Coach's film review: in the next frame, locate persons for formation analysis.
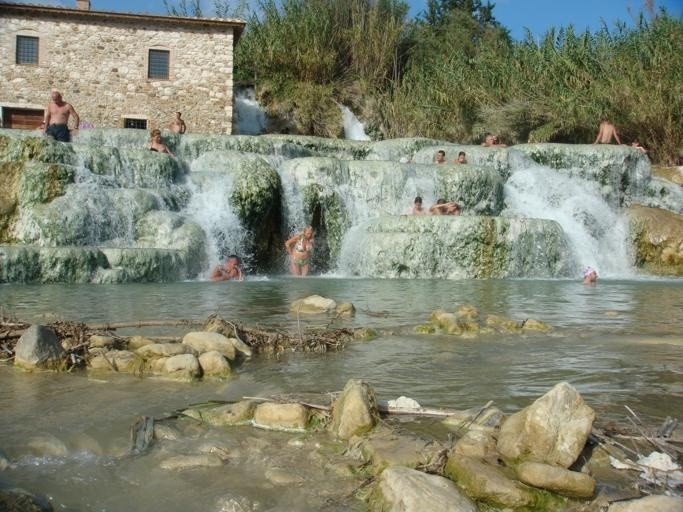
[449,151,466,165]
[583,266,596,284]
[169,112,186,134]
[210,255,242,283]
[434,151,448,165]
[283,226,314,277]
[410,197,426,216]
[43,91,79,142]
[480,132,508,148]
[591,112,621,145]
[629,138,648,154]
[428,198,456,215]
[145,130,176,157]
